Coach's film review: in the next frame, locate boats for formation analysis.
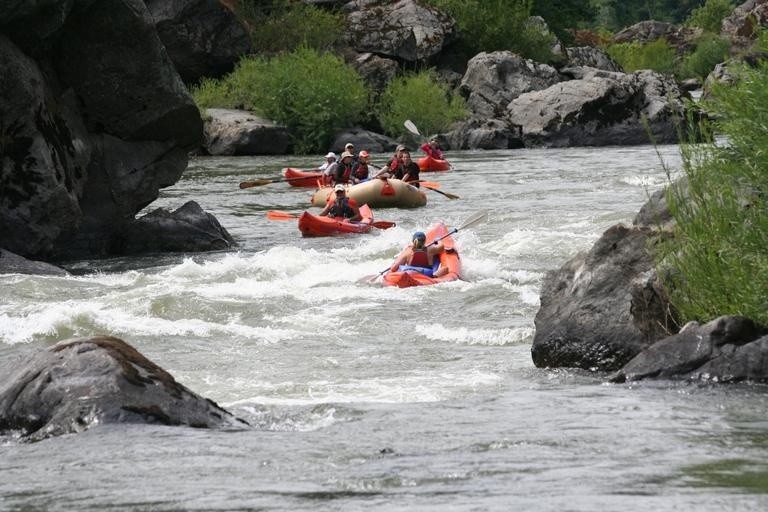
[297,202,372,233]
[284,169,335,187]
[382,222,462,288]
[416,154,450,172]
[309,179,428,211]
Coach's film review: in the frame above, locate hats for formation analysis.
[395,144,406,152]
[324,143,370,163]
[335,184,344,192]
[413,232,425,240]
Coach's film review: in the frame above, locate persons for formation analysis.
[391,232,445,274]
[325,142,419,188]
[421,141,442,161]
[318,184,361,222]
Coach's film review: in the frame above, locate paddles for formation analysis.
[406,181,441,189]
[381,177,395,195]
[368,162,458,198]
[357,209,488,285]
[267,210,396,229]
[404,120,452,168]
[241,174,324,189]
